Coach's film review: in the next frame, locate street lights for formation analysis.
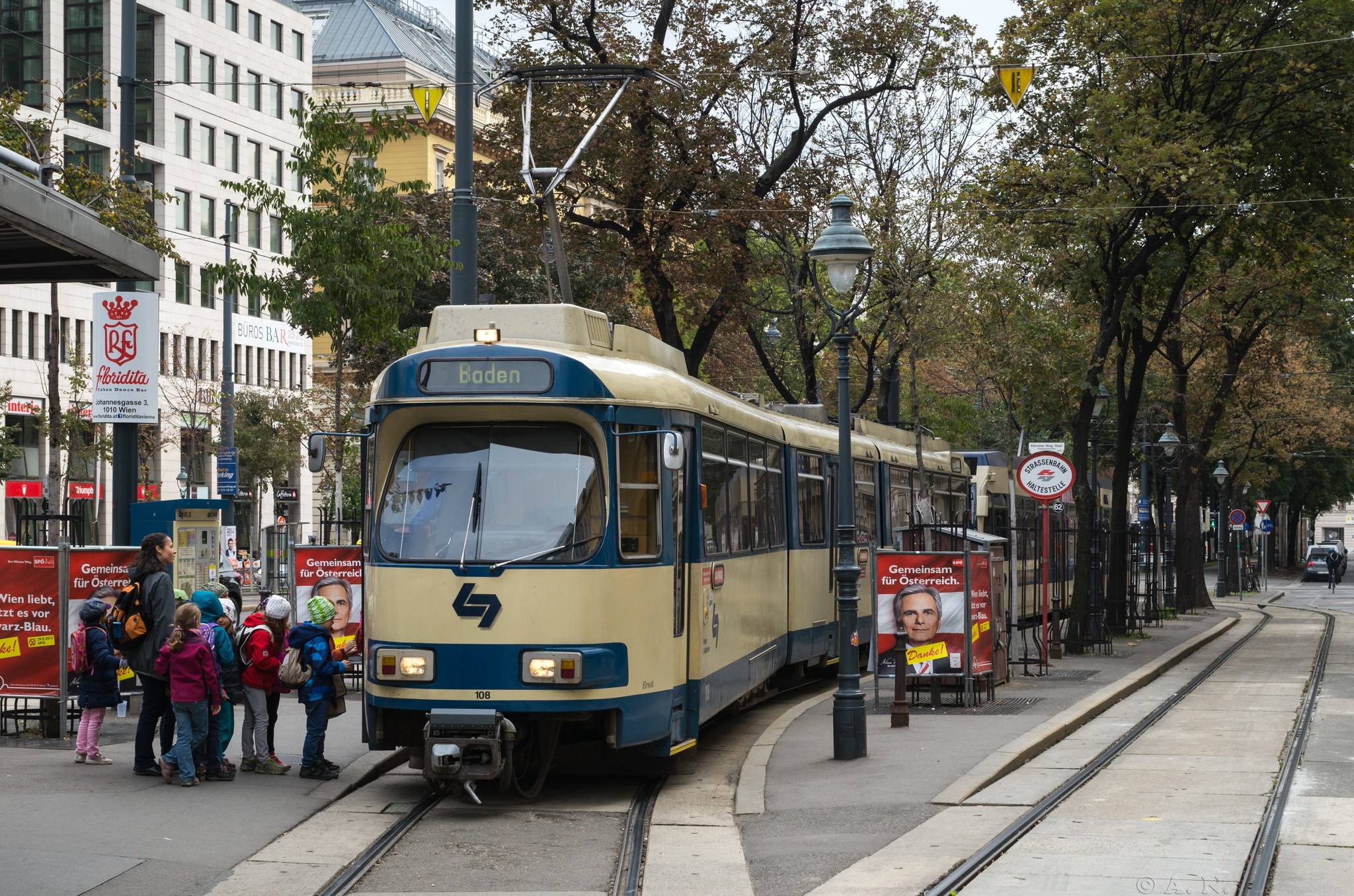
[809,194,878,760]
[1157,422,1181,608]
[1212,460,1230,597]
[1089,377,1111,642]
[1240,480,1252,591]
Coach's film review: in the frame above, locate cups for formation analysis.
[117,701,127,717]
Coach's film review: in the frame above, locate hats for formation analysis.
[79,597,112,620]
[218,598,235,623]
[266,594,291,619]
[201,581,229,598]
[306,595,337,624]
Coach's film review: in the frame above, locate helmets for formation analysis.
[1329,548,1335,552]
[260,597,271,609]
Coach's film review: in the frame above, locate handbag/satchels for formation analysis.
[327,674,346,720]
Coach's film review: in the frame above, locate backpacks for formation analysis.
[105,574,154,650]
[1330,551,1338,562]
[70,622,107,676]
[235,624,274,673]
[200,622,223,681]
[277,638,329,691]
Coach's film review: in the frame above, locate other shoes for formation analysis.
[299,763,339,780]
[135,765,173,777]
[74,753,86,762]
[1328,585,1331,588]
[85,753,113,765]
[317,761,339,773]
[133,761,159,771]
[323,759,340,772]
[181,777,200,787]
[268,750,291,769]
[205,768,235,782]
[222,758,236,767]
[197,762,206,776]
[159,757,174,784]
[220,764,237,773]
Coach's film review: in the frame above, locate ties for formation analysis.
[920,661,931,674]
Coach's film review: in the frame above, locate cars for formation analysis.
[1302,552,1342,583]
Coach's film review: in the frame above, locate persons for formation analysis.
[354,608,362,653]
[73,597,128,765]
[236,595,291,775]
[226,538,235,557]
[878,584,962,675]
[1329,528,1336,539]
[1239,554,1253,581]
[1326,548,1341,589]
[393,450,466,559]
[87,585,121,619]
[127,533,176,776]
[155,603,221,786]
[173,581,244,781]
[286,595,358,780]
[311,575,352,639]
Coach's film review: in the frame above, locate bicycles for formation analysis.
[1244,565,1261,593]
[1330,565,1338,594]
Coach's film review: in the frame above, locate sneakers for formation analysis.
[239,755,256,771]
[254,757,289,774]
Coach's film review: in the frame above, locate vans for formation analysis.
[1306,540,1348,576]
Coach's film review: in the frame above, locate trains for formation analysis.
[307,62,1115,806]
